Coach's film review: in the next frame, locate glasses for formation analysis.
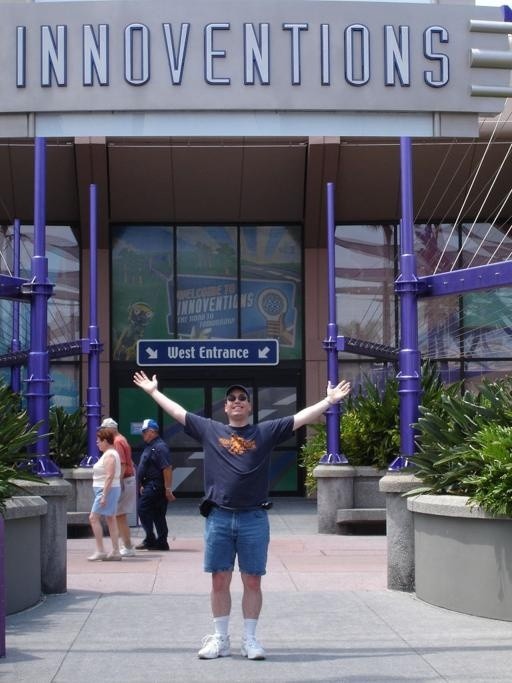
[227,394,248,402]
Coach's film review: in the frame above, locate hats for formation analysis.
[224,384,251,402]
[96,417,118,430]
[139,418,160,432]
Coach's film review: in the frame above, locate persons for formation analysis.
[134,419,176,550]
[101,417,137,557]
[133,369,351,660]
[87,429,122,561]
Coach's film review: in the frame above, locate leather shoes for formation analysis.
[135,539,156,549]
[147,540,170,551]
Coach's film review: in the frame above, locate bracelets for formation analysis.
[102,493,107,497]
[165,488,171,490]
[325,397,333,405]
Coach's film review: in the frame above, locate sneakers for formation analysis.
[196,632,232,658]
[87,552,107,561]
[120,545,136,557]
[102,552,123,562]
[239,635,266,660]
